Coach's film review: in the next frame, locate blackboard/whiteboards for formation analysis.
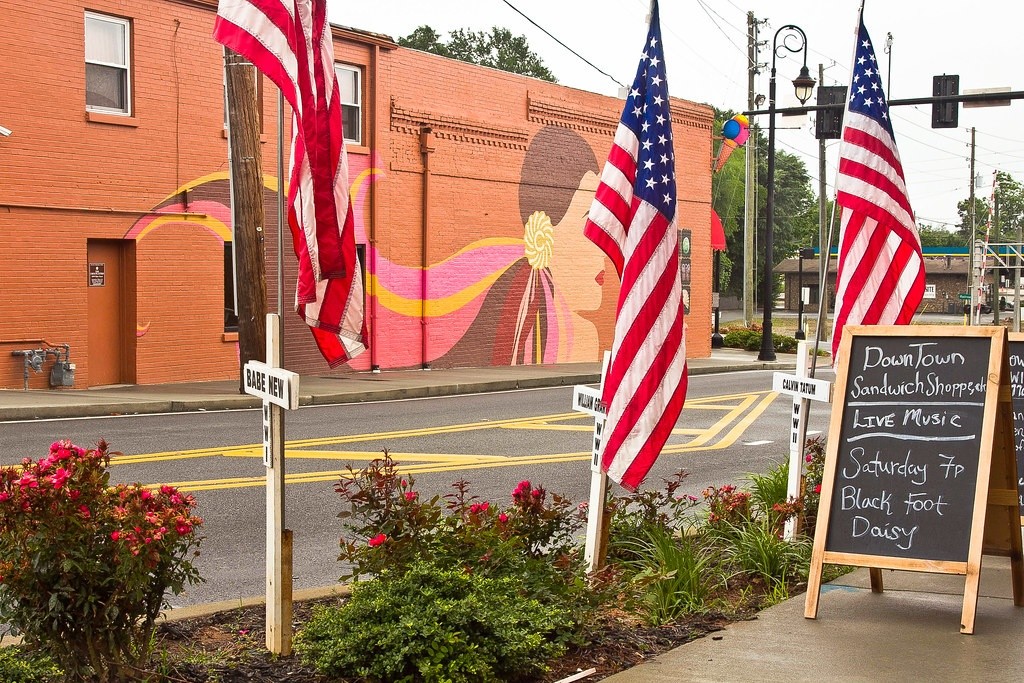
[802,325,1024,634]
[1005,325,1023,534]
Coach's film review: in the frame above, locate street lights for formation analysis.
[755,24,816,361]
[969,171,983,326]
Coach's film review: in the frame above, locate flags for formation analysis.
[213,0,370,370]
[584,0,691,495]
[830,5,928,373]
